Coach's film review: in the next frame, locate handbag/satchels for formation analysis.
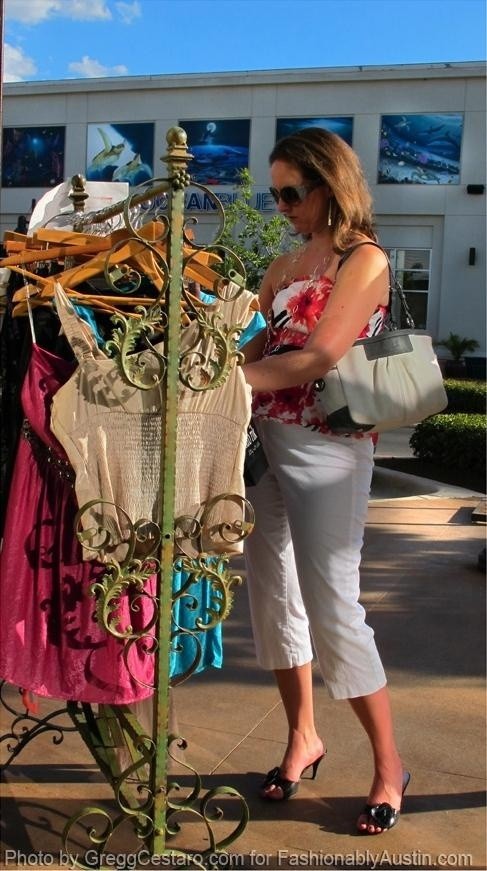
[316,329,449,435]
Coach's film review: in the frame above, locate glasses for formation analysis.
[268,179,323,204]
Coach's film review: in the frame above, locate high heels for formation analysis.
[356,769,410,835]
[260,745,327,798]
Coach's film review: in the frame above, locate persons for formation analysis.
[238,125,409,834]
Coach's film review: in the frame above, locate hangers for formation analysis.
[0,186,262,350]
